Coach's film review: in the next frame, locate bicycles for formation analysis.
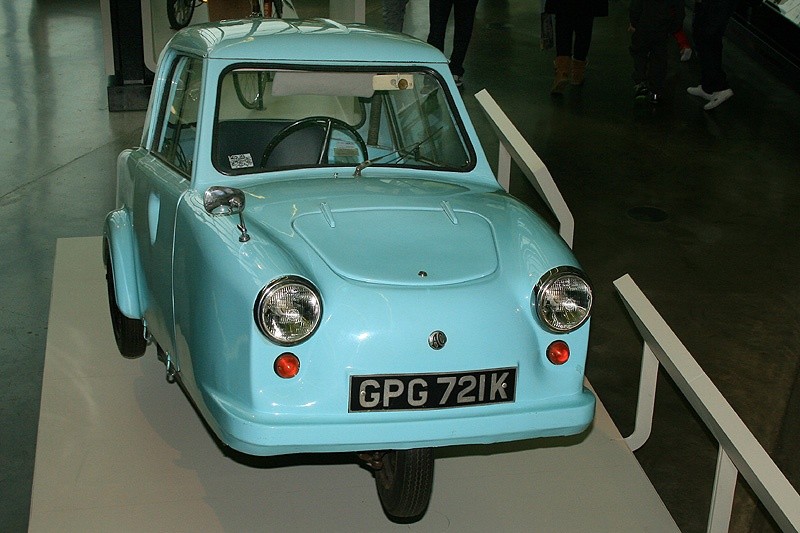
[167,0,282,111]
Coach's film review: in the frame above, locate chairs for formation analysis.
[211,119,327,173]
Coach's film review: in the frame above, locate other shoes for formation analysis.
[452,74,464,85]
[680,47,693,61]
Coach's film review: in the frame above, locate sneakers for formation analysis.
[634,86,665,104]
[687,84,710,99]
[703,88,733,110]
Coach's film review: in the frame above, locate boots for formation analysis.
[550,56,570,93]
[569,54,590,87]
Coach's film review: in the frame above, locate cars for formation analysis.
[102,20,597,525]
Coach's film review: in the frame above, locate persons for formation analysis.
[687,0,733,110]
[675,29,691,62]
[627,0,685,103]
[545,0,609,88]
[427,0,479,86]
